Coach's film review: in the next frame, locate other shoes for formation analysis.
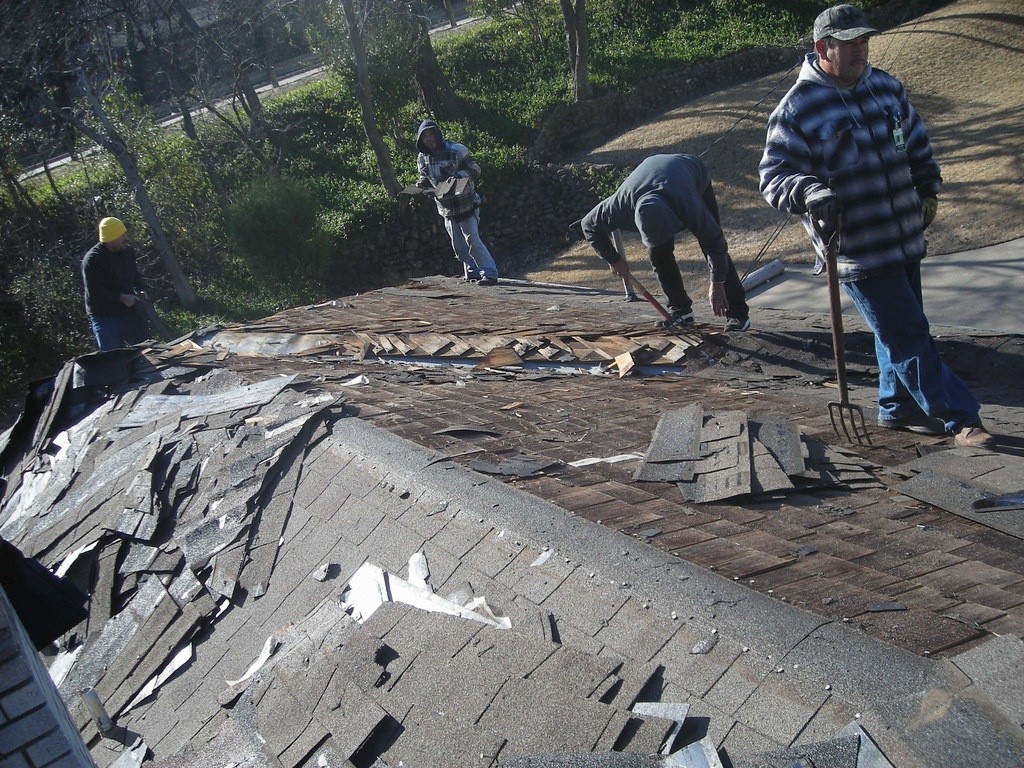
[476,276,497,285]
[955,422,997,449]
[462,275,481,282]
[878,416,946,436]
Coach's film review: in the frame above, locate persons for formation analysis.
[757,5,995,450]
[415,119,498,286]
[81,217,149,351]
[581,153,751,332]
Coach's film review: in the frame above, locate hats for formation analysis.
[813,5,879,41]
[99,217,128,243]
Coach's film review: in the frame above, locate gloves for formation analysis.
[921,195,938,224]
[802,183,836,220]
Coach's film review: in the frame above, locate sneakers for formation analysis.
[724,314,751,332]
[654,309,695,327]
